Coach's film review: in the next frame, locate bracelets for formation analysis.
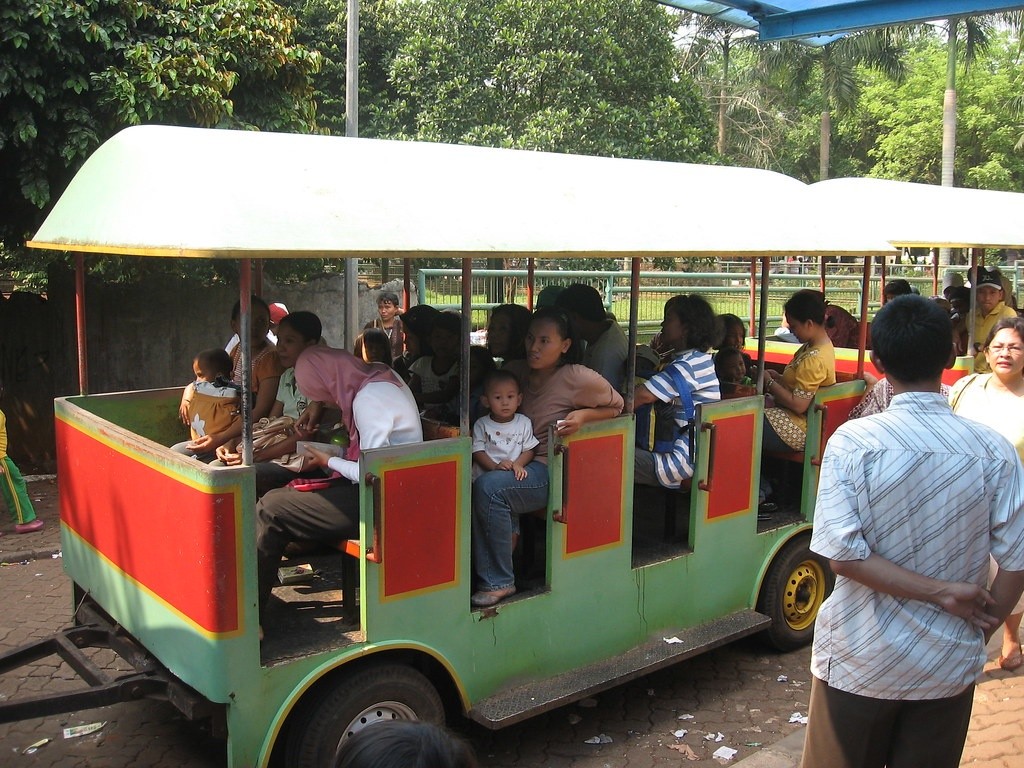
[764,378,774,388]
[180,397,192,404]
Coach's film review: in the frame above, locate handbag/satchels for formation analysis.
[189,393,242,444]
[236,415,296,454]
[635,398,678,453]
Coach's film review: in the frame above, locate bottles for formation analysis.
[330,430,350,447]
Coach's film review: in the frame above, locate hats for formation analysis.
[988,266,1001,274]
[910,285,920,296]
[977,272,1002,292]
[942,272,963,293]
[536,286,569,308]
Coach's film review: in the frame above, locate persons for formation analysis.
[353,328,393,366]
[170,295,282,464]
[714,349,756,388]
[393,283,629,607]
[255,345,424,640]
[713,314,752,379]
[947,316,1024,671]
[749,291,836,513]
[788,256,806,274]
[193,348,234,383]
[781,289,859,349]
[847,374,951,420]
[207,311,342,501]
[0,410,44,536]
[225,302,289,355]
[798,294,1024,768]
[883,265,1018,373]
[363,293,407,357]
[619,295,727,489]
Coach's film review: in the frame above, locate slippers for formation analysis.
[758,513,772,521]
[760,502,778,511]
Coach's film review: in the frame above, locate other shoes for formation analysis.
[1000,639,1022,671]
[15,519,44,534]
[470,584,516,606]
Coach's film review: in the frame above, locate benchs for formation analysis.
[307,359,868,638]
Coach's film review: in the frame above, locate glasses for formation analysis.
[987,345,1024,355]
